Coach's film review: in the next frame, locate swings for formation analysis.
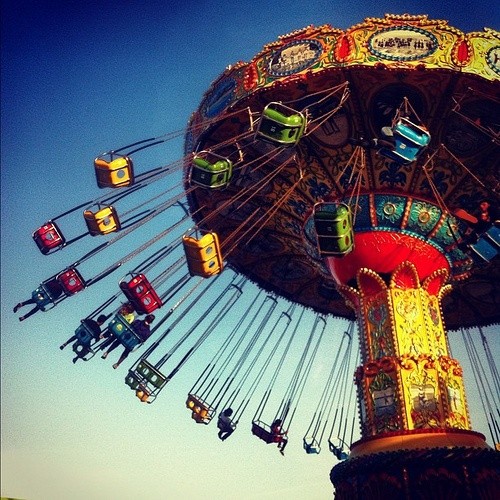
[29,76,500,460]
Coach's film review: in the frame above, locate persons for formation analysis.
[360,116,413,149]
[60,315,107,364]
[13,279,62,321]
[217,407,236,441]
[102,314,155,369]
[271,419,288,455]
[100,302,135,350]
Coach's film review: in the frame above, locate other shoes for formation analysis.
[280,450,284,456]
[73,359,76,364]
[13,303,22,313]
[101,353,106,359]
[60,345,64,350]
[19,316,24,321]
[112,364,118,369]
